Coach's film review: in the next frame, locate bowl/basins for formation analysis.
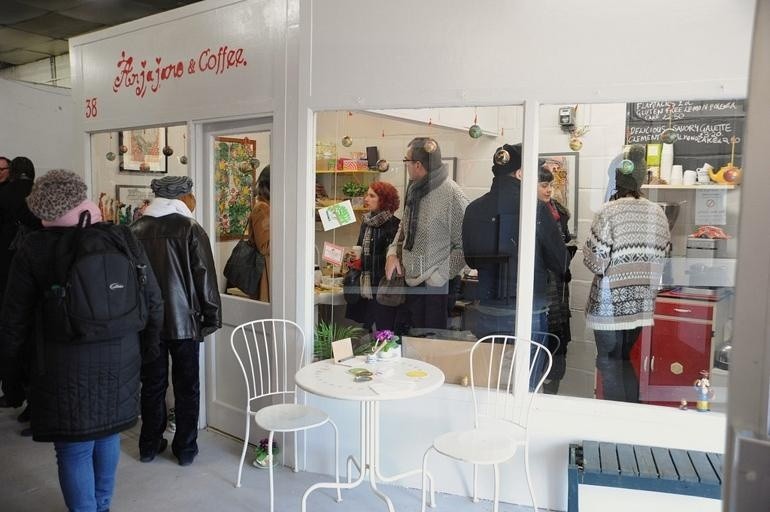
[322,275,343,286]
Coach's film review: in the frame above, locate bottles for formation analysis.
[314,266,322,287]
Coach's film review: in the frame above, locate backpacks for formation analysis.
[40,221,148,342]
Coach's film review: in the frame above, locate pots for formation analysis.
[655,201,681,230]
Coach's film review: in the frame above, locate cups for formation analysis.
[378,346,392,358]
[684,169,697,185]
[660,142,674,185]
[696,167,711,183]
[352,246,363,262]
[670,164,683,185]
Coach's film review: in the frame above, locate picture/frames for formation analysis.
[118,127,168,173]
[215,137,256,241]
[115,184,158,207]
[403,156,457,209]
[538,151,579,239]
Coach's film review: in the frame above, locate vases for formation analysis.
[252,455,279,469]
[379,344,403,361]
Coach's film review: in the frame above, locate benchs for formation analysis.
[567,439,725,512]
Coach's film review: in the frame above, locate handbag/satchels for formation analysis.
[376,264,407,307]
[343,268,362,305]
[223,239,267,294]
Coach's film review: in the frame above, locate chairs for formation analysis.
[230,319,342,512]
[420,335,552,512]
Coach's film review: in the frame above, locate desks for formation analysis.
[295,354,446,512]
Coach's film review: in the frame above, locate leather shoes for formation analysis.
[141,439,167,462]
[1,395,32,436]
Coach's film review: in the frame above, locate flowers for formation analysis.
[371,329,399,350]
[253,437,280,462]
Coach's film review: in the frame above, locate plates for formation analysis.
[376,352,398,360]
[319,199,343,206]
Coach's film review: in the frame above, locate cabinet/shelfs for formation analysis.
[593,287,731,411]
[313,168,380,215]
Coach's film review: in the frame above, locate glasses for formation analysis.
[405,158,415,163]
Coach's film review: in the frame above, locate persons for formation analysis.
[0,154,269,512]
[342,137,470,335]
[462,141,578,394]
[583,145,672,404]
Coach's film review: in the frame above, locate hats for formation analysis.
[615,144,650,191]
[26,169,87,220]
[492,142,522,176]
[151,175,193,199]
